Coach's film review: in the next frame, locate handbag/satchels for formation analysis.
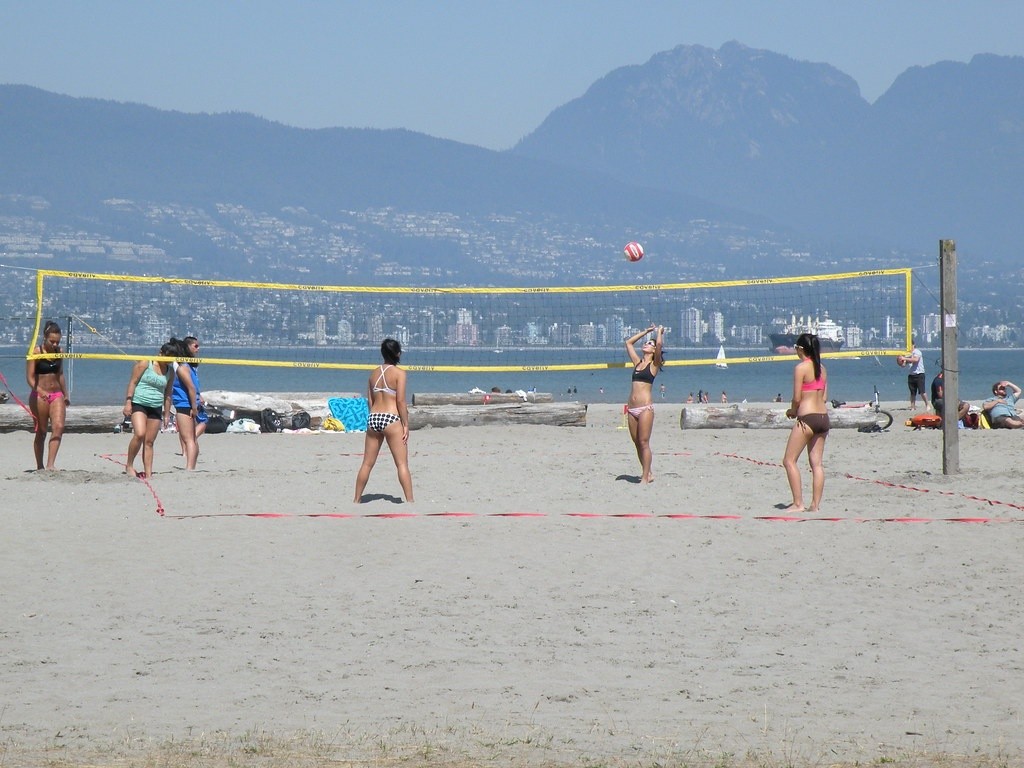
[205,415,227,433]
[261,408,283,432]
[322,416,344,431]
[292,410,312,430]
[226,418,261,434]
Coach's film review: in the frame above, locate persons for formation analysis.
[687,392,693,403]
[172,340,200,471]
[567,387,571,393]
[721,391,727,402]
[660,384,665,398]
[573,386,578,393]
[982,381,1024,428]
[932,372,970,421]
[173,337,208,456]
[600,388,604,393]
[625,322,665,483]
[783,334,830,513]
[123,338,182,480]
[704,392,709,403]
[901,341,930,412]
[696,389,702,403]
[776,394,781,402]
[353,339,414,504]
[26,321,70,469]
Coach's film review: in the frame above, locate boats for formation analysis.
[766,332,843,356]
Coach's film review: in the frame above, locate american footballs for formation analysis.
[896,355,904,365]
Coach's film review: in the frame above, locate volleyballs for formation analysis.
[623,241,644,262]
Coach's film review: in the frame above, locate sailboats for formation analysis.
[716,345,729,370]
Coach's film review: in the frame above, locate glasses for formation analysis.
[190,345,198,348]
[644,342,655,347]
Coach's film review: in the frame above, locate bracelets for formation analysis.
[126,396,132,400]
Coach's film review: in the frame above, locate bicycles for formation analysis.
[831,384,894,431]
